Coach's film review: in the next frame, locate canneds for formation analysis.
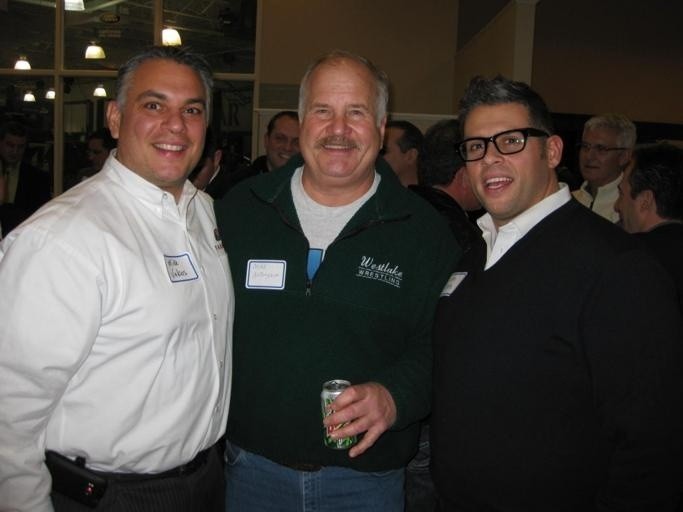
[321,379,357,450]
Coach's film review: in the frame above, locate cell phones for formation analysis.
[43,451,107,508]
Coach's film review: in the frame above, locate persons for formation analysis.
[250,109,301,174]
[81,127,117,181]
[190,130,235,198]
[380,120,423,187]
[613,137,683,303]
[0,46,237,512]
[568,111,639,225]
[405,117,486,232]
[214,47,463,511]
[0,119,52,240]
[428,74,682,511]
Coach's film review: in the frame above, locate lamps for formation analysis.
[12,25,182,102]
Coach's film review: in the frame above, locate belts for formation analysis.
[101,449,209,483]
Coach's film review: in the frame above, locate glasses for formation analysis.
[574,141,632,157]
[455,128,548,163]
